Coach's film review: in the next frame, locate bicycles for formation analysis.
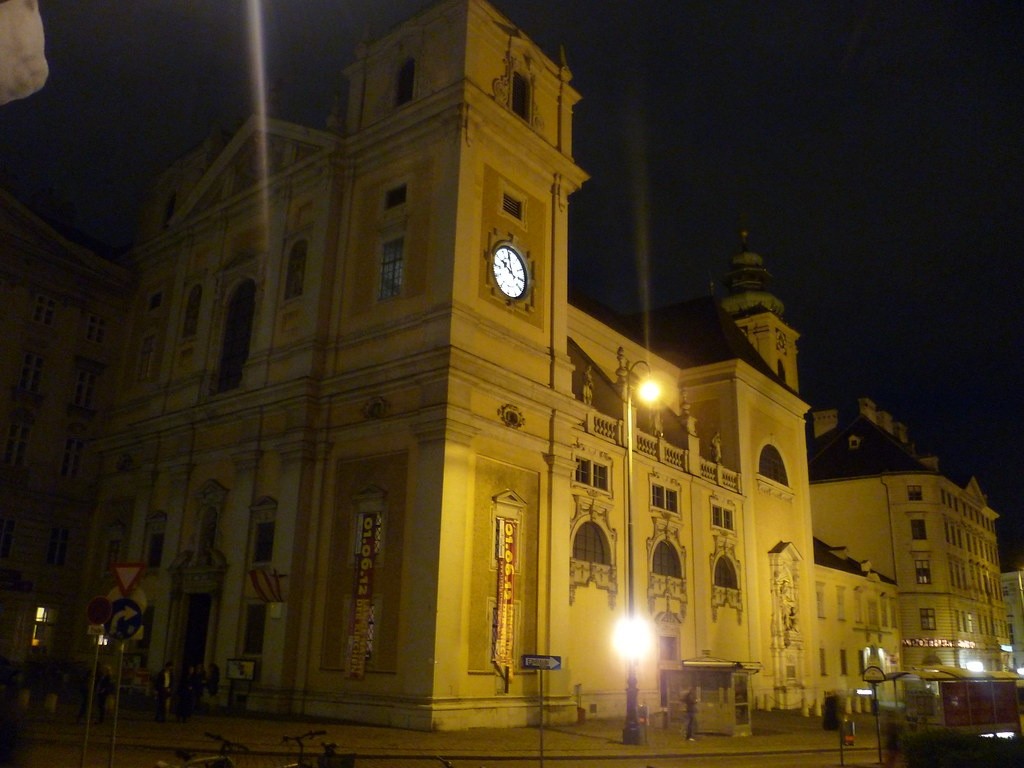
[277,729,357,768]
[153,729,251,768]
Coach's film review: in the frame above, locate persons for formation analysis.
[683,688,699,743]
[77,659,112,725]
[156,661,219,723]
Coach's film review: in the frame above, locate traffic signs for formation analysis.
[521,654,562,671]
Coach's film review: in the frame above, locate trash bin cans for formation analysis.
[823,696,846,730]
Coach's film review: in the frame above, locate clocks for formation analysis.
[491,240,531,304]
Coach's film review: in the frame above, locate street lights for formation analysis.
[614,359,663,745]
